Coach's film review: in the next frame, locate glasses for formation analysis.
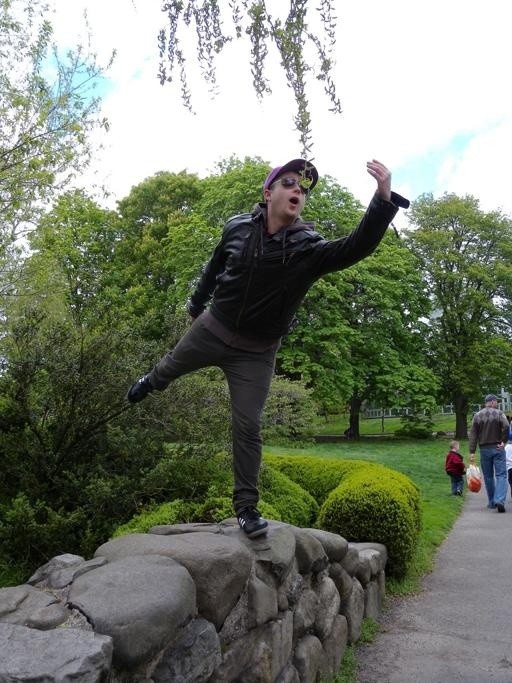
[269,176,310,194]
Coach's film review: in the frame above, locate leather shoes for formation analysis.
[497,502,506,512]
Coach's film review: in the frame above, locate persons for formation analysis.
[446,441,465,496]
[469,394,509,512]
[127,158,410,538]
[505,416,512,497]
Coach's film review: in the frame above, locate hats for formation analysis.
[485,393,502,402]
[264,159,318,189]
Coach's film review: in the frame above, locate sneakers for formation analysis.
[127,372,155,403]
[237,504,269,538]
[457,489,461,495]
